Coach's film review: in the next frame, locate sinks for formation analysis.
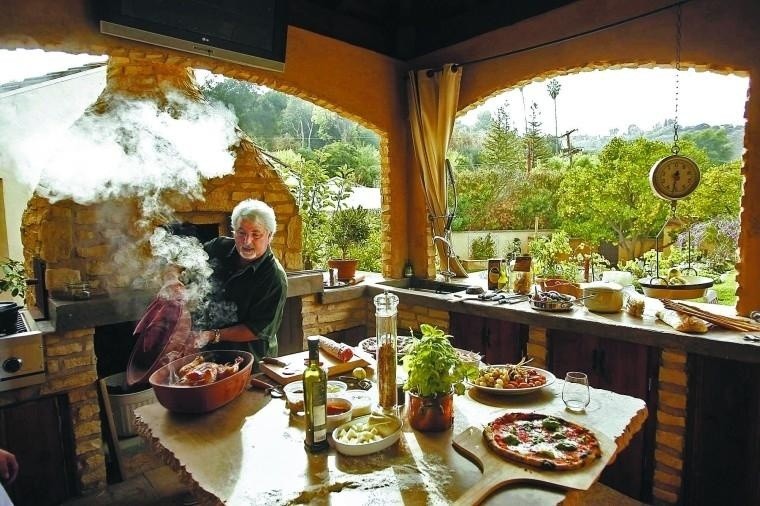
[375,278,471,296]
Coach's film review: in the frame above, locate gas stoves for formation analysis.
[0,307,46,393]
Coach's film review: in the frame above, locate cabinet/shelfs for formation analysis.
[547,327,657,504]
[448,310,527,368]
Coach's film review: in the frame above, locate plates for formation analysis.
[359,334,416,358]
[50,291,105,300]
[467,364,557,396]
[450,348,480,364]
[330,418,405,457]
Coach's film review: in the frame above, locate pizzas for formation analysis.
[483,413,601,471]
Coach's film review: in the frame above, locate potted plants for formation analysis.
[327,201,370,280]
[400,321,482,433]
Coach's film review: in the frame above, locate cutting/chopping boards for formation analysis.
[259,346,369,384]
[449,421,619,506]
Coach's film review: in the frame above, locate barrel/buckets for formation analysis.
[102,371,160,437]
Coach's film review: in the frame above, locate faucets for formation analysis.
[432,236,451,282]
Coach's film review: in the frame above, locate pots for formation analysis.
[0,300,24,329]
[530,291,595,310]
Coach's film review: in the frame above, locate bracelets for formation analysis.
[211,329,220,344]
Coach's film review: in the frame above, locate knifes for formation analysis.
[476,290,528,304]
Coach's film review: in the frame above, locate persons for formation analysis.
[0,448,19,506]
[156,197,288,375]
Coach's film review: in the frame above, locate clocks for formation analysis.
[648,0,703,238]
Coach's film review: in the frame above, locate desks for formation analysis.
[132,335,648,505]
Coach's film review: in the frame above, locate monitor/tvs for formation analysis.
[100,0,289,72]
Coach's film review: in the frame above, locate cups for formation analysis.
[562,371,591,413]
[64,281,91,297]
[330,268,339,286]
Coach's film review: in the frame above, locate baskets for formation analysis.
[540,278,583,300]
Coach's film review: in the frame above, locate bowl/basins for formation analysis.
[123,296,192,389]
[582,280,624,314]
[320,377,375,429]
[154,348,256,416]
[284,380,310,420]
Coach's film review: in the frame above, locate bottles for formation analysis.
[403,263,414,278]
[302,334,332,452]
[498,259,511,293]
[510,260,534,296]
[304,255,313,270]
